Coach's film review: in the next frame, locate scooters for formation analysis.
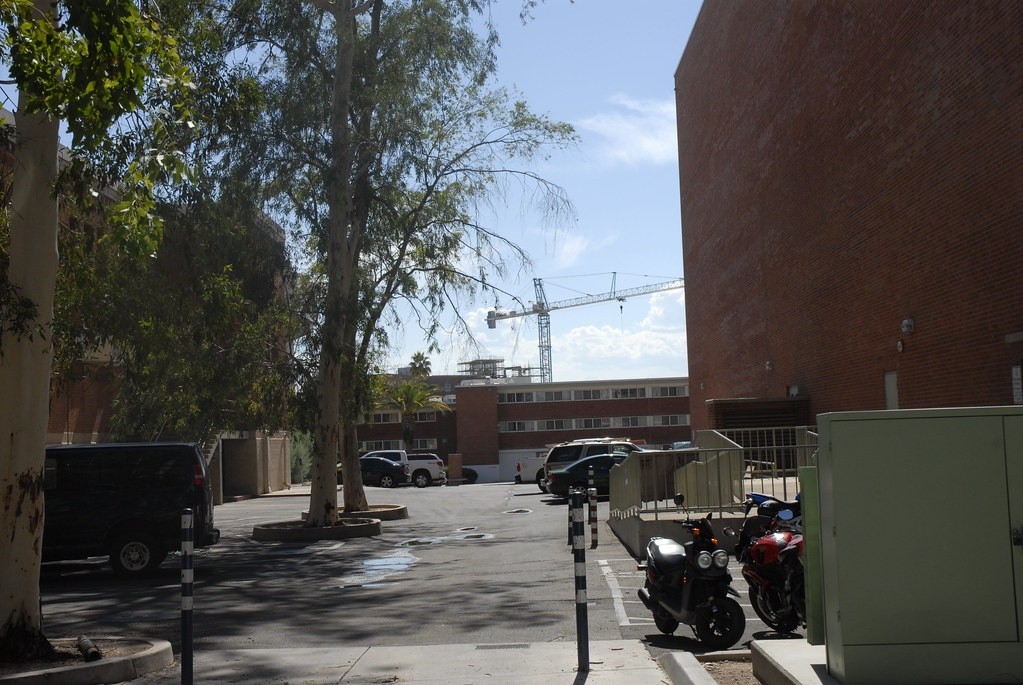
[637,493,745,650]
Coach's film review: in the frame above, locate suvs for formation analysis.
[540,437,662,487]
[407,454,446,488]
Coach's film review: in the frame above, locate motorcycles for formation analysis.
[723,491,807,634]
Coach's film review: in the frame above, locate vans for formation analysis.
[42,442,220,577]
[336,450,409,475]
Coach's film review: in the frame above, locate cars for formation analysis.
[545,451,629,501]
[668,441,692,450]
[336,457,412,488]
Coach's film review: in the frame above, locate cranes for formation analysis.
[484,272,685,383]
